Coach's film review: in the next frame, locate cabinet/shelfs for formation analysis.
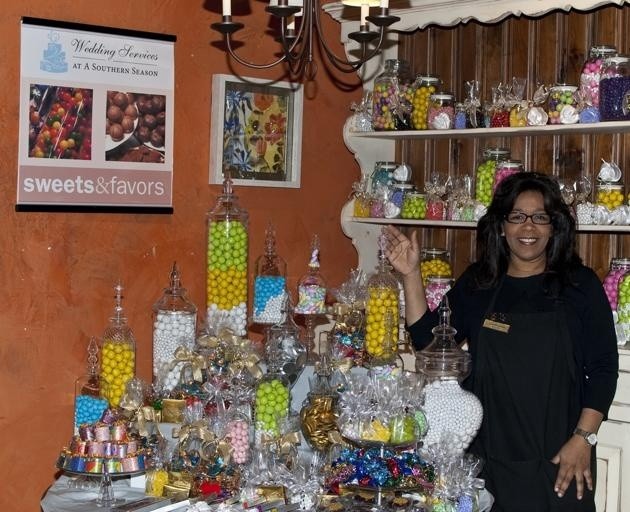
[333,109,630,512]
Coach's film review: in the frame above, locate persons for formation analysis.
[380,171,619,512]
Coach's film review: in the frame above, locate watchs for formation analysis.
[573,427,599,447]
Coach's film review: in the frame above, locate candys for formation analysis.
[371,58,630,131]
[57,161,630,512]
[29,89,92,160]
[105,90,165,148]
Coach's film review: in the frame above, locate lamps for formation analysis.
[210,0,401,80]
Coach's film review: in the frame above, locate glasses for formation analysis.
[505,210,557,225]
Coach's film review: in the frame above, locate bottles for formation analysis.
[371,43,630,131]
[417,246,630,348]
[72,168,484,462]
[351,147,626,225]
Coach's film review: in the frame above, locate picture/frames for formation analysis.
[209,73,304,188]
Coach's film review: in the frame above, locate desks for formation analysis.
[39,363,494,512]
